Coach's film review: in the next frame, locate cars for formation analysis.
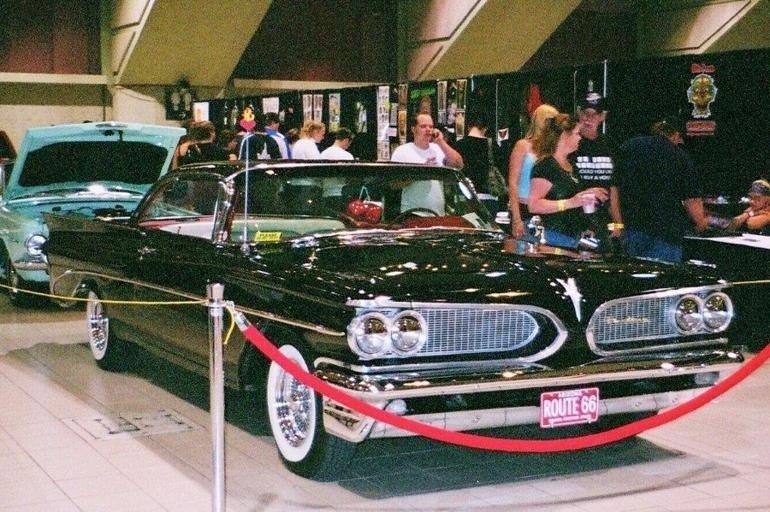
[0,122,191,310]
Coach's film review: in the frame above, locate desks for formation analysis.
[678,229,769,354]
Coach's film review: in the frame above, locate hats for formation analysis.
[578,91,607,114]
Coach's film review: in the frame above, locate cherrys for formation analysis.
[347,186,382,223]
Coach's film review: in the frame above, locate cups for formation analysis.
[580,190,598,215]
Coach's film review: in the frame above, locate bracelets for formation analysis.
[607,223,624,230]
[558,200,565,211]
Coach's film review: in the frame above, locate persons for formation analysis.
[390,114,500,218]
[730,179,770,233]
[509,105,560,240]
[170,112,354,215]
[527,114,609,248]
[567,93,625,252]
[610,117,708,264]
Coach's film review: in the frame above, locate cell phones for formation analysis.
[432,133,438,138]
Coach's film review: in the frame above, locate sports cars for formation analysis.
[40,159,746,485]
